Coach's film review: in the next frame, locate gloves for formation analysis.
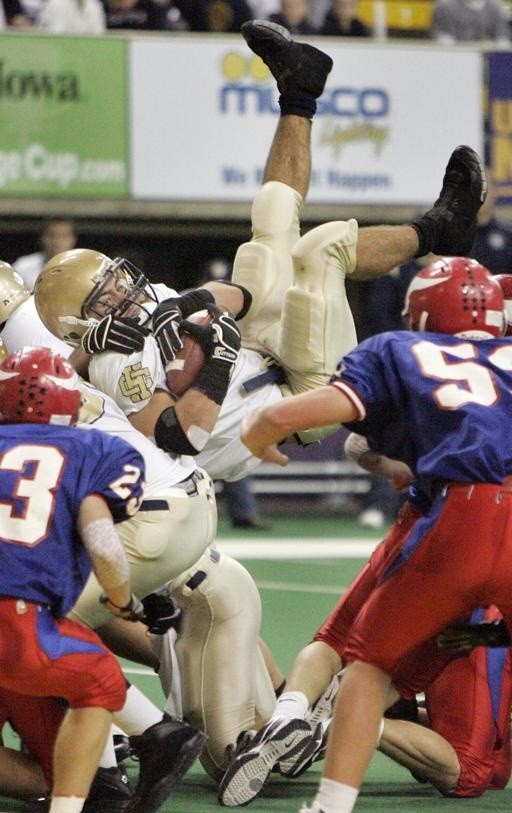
[140,594,183,634]
[152,290,214,365]
[99,591,145,622]
[180,302,241,406]
[80,314,148,355]
[438,618,509,659]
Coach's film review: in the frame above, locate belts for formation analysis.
[243,352,288,390]
[140,469,206,509]
[189,548,221,590]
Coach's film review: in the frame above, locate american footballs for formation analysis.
[165,308,216,395]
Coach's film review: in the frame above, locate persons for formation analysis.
[0,218,511,812]
[32,16,492,485]
[1,1,509,40]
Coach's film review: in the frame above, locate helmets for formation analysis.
[0,346,81,427]
[34,248,160,349]
[0,260,31,324]
[401,256,512,343]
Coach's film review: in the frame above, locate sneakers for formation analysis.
[241,20,333,99]
[81,667,349,812]
[424,145,487,256]
